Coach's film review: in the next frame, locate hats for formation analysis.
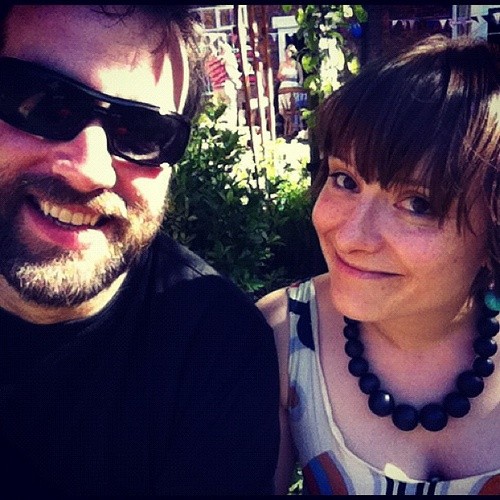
[285,44,298,57]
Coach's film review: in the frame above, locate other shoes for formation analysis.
[286,135,291,144]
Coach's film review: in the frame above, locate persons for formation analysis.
[278,44,304,139]
[255,35,500,497]
[0,0,285,500]
[205,36,242,129]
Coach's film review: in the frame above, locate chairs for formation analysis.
[278,87,308,137]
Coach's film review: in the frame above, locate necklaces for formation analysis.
[342,263,499,432]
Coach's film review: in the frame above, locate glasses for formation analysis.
[0,56,192,167]
